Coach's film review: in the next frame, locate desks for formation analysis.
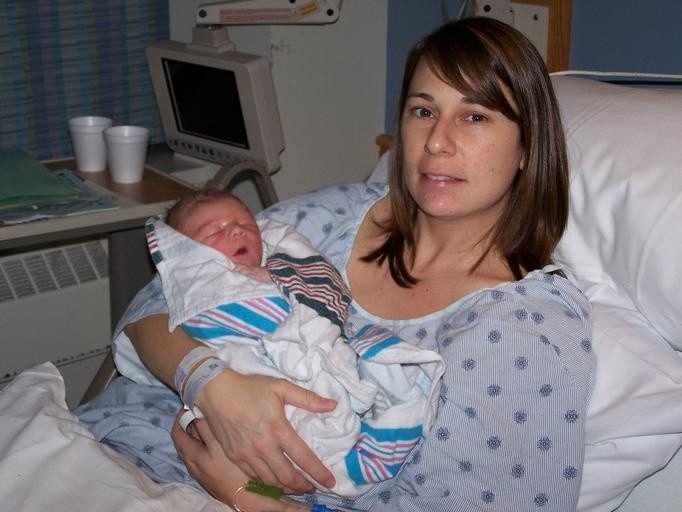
[0,150,201,413]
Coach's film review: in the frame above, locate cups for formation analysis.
[66,115,150,185]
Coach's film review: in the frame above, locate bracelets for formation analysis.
[175,346,220,405]
[183,358,229,419]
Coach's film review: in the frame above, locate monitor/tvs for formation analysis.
[146,46,278,177]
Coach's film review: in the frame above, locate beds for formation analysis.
[0,70,682,512]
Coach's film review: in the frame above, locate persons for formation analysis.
[146,189,446,497]
[76,16,593,512]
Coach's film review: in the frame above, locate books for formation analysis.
[1,146,78,211]
[1,170,122,225]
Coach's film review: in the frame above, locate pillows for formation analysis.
[365,74,682,356]
